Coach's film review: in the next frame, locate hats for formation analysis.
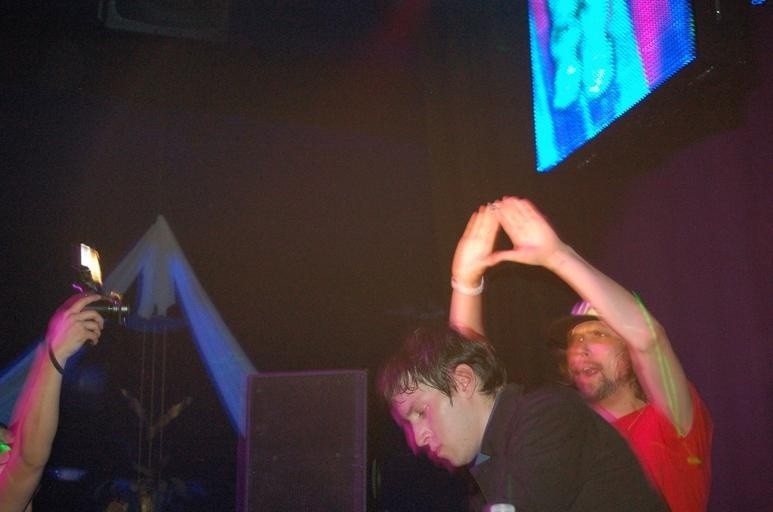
[541,296,603,345]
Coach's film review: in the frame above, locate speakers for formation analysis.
[242,370,397,511]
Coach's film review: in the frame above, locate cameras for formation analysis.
[69,279,130,327]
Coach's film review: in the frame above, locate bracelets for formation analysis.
[449,275,485,296]
[46,340,66,376]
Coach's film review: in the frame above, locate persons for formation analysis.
[379,320,672,512]
[0,291,105,512]
[447,195,716,511]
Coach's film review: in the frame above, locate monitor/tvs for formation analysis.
[523,0,722,187]
